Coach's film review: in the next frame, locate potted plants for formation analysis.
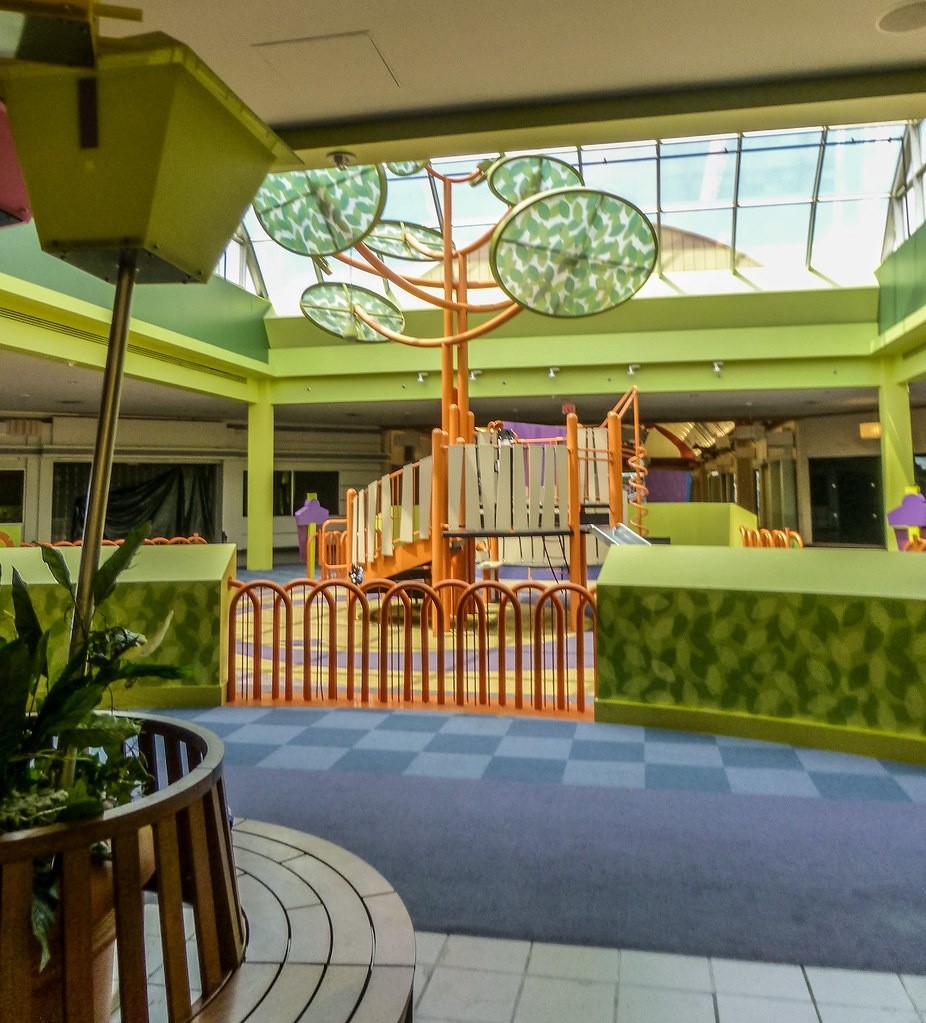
[0,519,189,1023]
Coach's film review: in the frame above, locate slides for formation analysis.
[591,522,656,549]
